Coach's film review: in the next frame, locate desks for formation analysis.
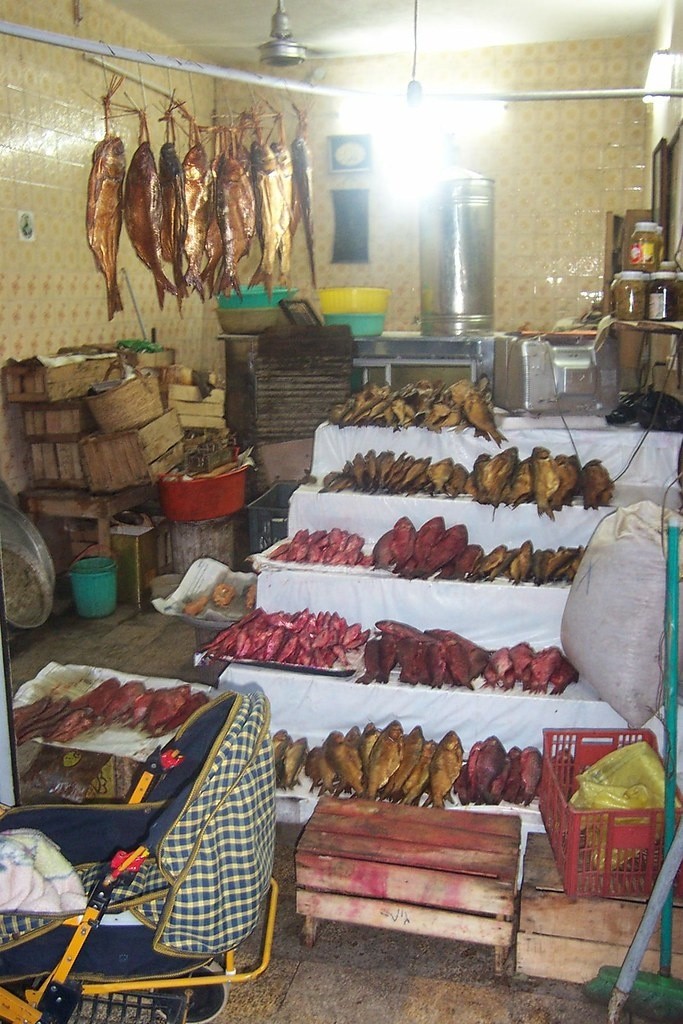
[17,484,157,547]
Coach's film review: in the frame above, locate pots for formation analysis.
[0,500,55,628]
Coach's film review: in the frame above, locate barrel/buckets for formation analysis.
[67,543,118,618]
[420,178,496,337]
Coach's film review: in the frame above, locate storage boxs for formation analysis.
[20,399,97,443]
[79,408,188,488]
[87,342,176,383]
[110,525,158,604]
[2,356,123,403]
[30,442,88,489]
[536,727,683,898]
[515,832,683,985]
[244,480,298,570]
[161,383,225,429]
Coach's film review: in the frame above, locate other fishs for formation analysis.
[86,135,126,321]
[172,135,316,320]
[121,142,182,310]
[157,143,186,265]
[183,144,214,294]
[194,606,580,696]
[318,449,616,523]
[14,677,574,810]
[268,516,587,586]
[327,377,509,449]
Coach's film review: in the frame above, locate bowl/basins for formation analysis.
[158,466,247,521]
[321,314,386,337]
[211,308,286,335]
[317,288,394,314]
[216,284,299,308]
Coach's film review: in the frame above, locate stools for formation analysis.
[288,795,523,975]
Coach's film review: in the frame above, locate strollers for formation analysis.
[0,682,281,1024]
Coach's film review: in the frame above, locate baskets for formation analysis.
[84,358,164,432]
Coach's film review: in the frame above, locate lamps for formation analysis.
[256,0,308,67]
[406,0,423,108]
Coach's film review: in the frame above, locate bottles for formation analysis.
[611,222,683,323]
